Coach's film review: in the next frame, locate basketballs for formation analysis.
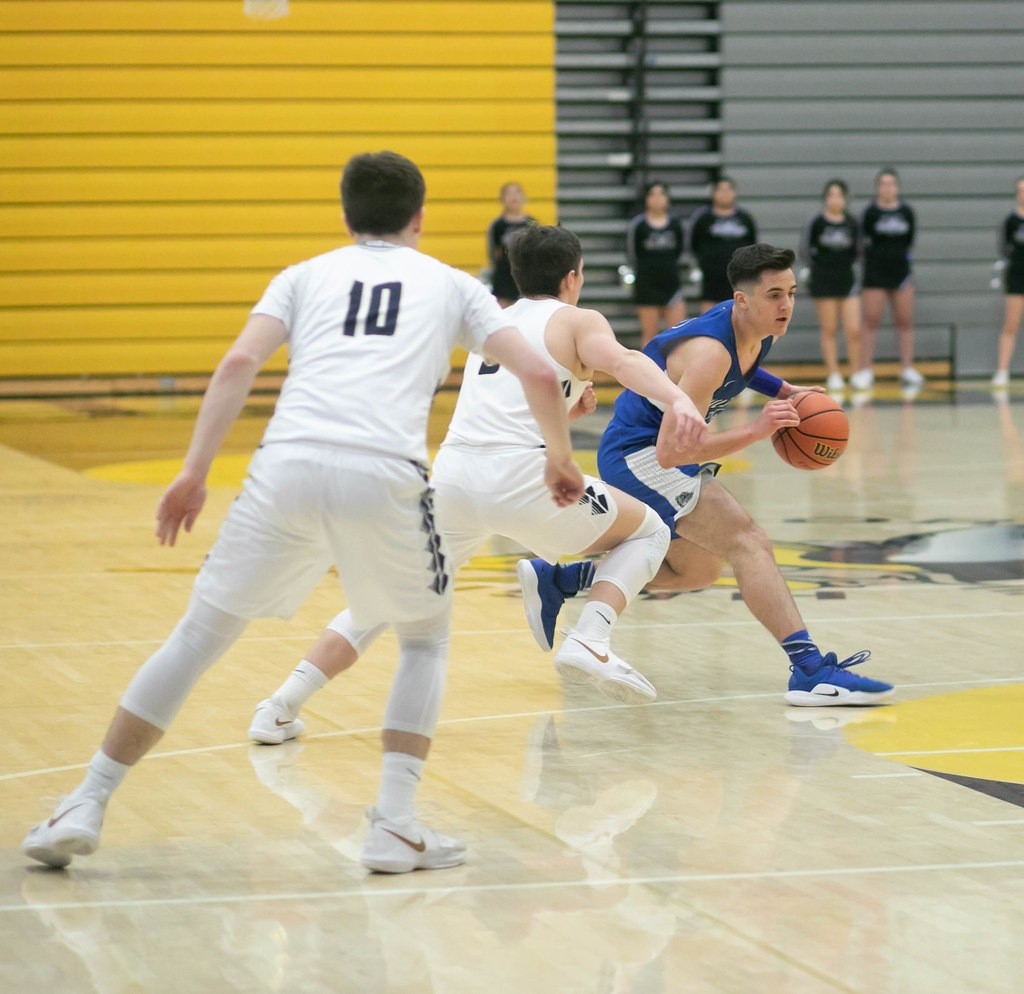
[768,390,851,471]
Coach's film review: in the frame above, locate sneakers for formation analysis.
[247,692,305,745]
[18,788,112,869]
[515,557,570,653]
[359,803,468,874]
[783,649,897,707]
[553,624,658,705]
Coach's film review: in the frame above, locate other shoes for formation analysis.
[990,389,1008,403]
[850,369,873,389]
[825,372,846,389]
[827,393,846,405]
[898,368,925,384]
[991,367,1010,387]
[850,390,874,407]
[898,385,923,401]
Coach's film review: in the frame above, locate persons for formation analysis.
[248,168,924,743]
[991,181,1024,388]
[20,150,583,872]
